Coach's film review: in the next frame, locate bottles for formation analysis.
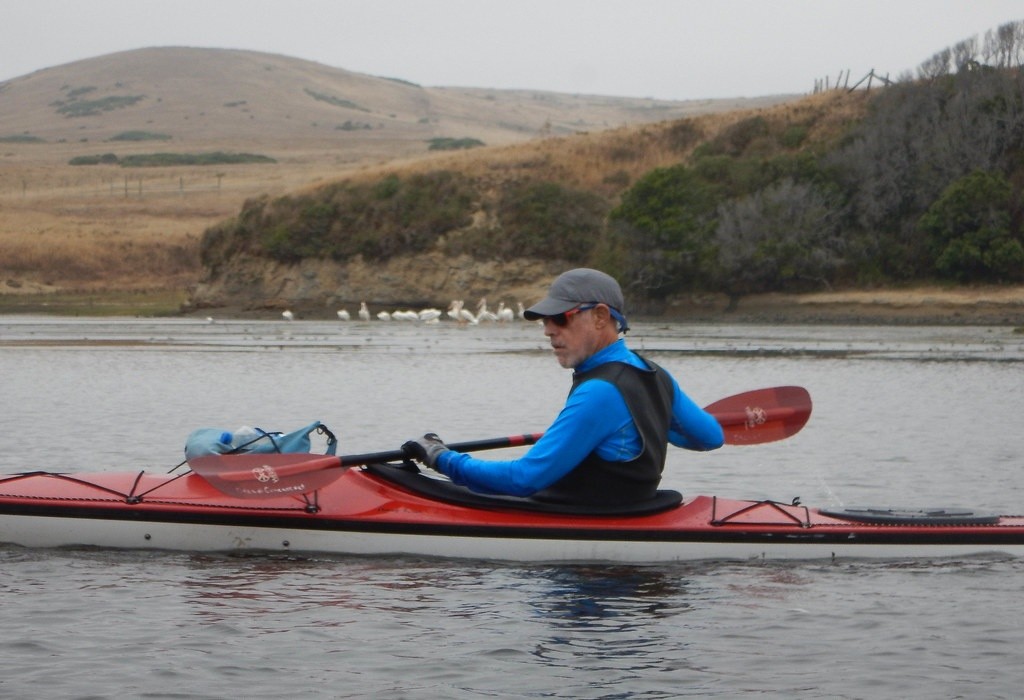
[220,425,286,450]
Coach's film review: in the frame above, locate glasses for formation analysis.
[540,303,595,327]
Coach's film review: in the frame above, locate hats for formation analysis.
[523,268,623,320]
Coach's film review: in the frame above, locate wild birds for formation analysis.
[516,302,525,320]
[206,317,216,325]
[281,310,294,321]
[447,297,514,325]
[358,301,370,323]
[392,310,418,321]
[376,311,391,322]
[419,308,442,323]
[337,309,351,321]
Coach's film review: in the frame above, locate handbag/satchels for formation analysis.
[185,420,338,462]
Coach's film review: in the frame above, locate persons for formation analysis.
[402,268,724,500]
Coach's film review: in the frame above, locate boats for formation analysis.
[1,463,1024,560]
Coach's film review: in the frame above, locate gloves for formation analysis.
[401,433,450,471]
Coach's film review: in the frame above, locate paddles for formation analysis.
[184,385,813,500]
